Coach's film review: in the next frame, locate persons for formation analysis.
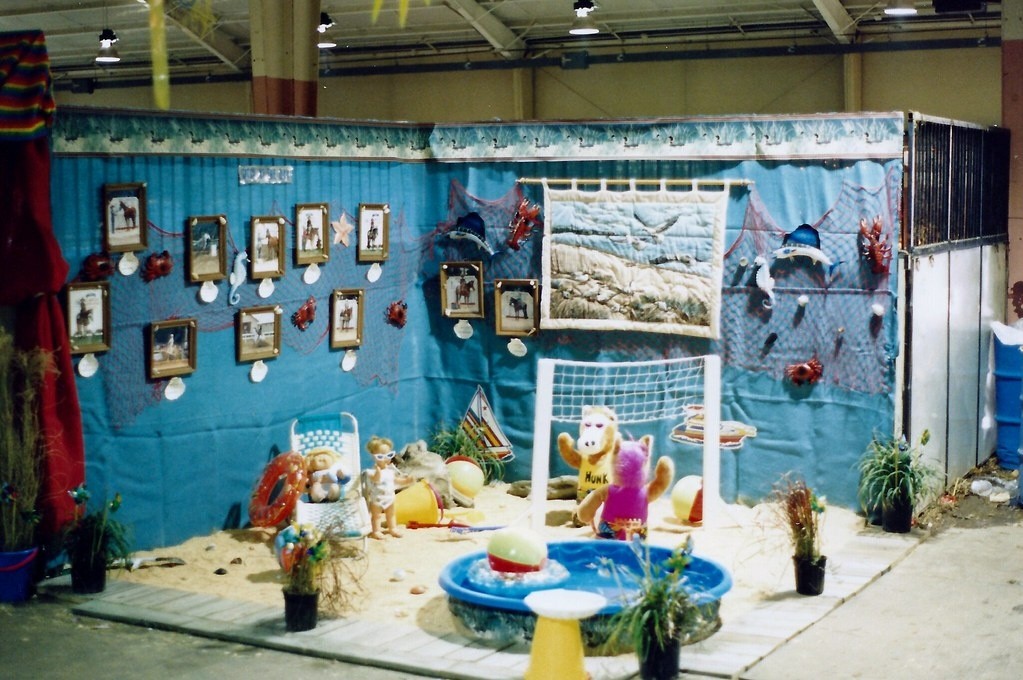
[301,445,349,505]
[360,435,405,539]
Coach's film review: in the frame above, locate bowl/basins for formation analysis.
[439,540,732,617]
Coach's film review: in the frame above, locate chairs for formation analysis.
[288,411,373,560]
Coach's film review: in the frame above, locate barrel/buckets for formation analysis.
[395,477,444,525]
[994,332,1023,471]
[0,547,39,601]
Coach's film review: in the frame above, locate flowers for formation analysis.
[744,470,829,563]
[848,422,951,528]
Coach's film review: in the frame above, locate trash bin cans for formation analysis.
[992,332,1023,470]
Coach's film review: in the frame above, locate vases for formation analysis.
[881,496,913,533]
[791,554,828,596]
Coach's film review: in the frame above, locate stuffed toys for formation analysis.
[557,405,675,542]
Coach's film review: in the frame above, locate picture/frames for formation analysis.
[495,279,538,337]
[147,317,197,380]
[358,203,389,262]
[187,214,227,283]
[237,304,281,363]
[330,288,363,348]
[250,215,285,279]
[100,182,148,254]
[65,282,111,354]
[440,261,484,319]
[294,203,329,265]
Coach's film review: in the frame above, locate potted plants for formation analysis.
[280,495,371,632]
[0,324,62,607]
[57,507,135,594]
[594,528,727,680]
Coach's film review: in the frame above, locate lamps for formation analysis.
[317,12,337,48]
[568,2,600,35]
[884,0,917,15]
[95,27,120,62]
